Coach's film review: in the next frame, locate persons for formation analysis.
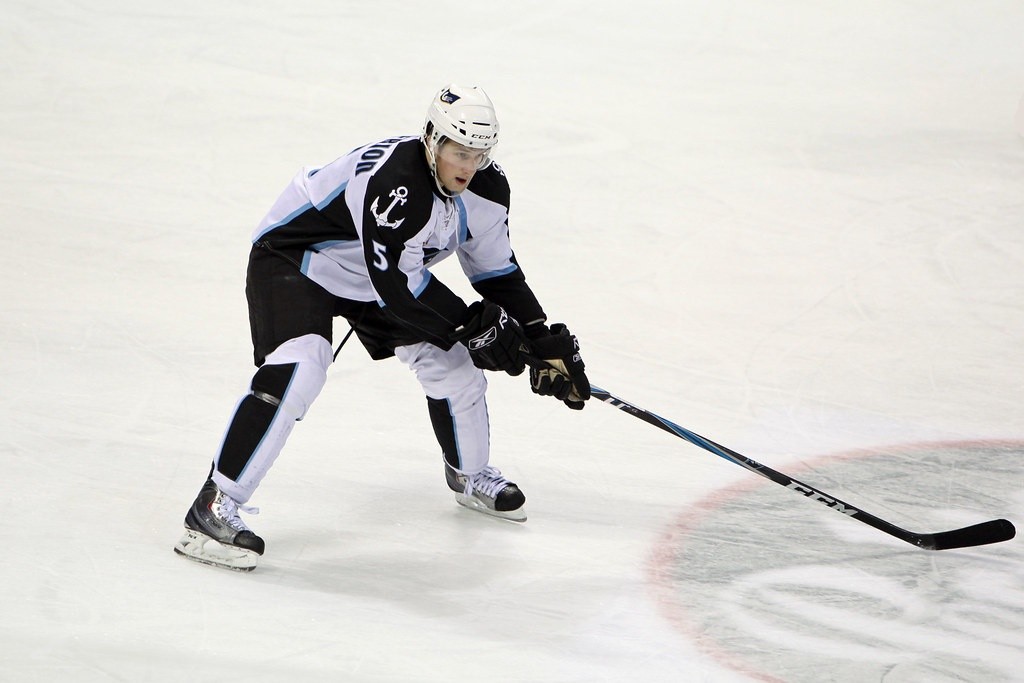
[173,81,592,574]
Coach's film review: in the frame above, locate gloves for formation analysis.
[457,299,527,377]
[523,322,591,411]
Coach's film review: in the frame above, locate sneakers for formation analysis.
[173,479,265,574]
[442,455,528,523]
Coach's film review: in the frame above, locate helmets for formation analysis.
[420,80,501,164]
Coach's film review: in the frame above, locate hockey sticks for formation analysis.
[519,350,1016,552]
